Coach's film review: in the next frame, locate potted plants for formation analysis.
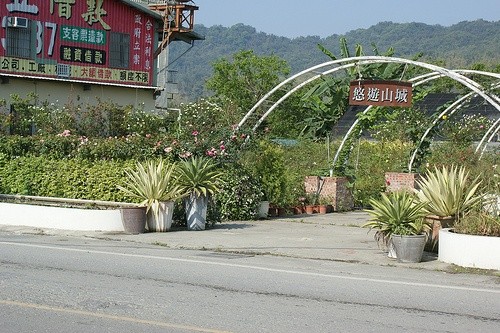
[269,202,294,216]
[295,192,332,214]
[392,227,427,263]
[116,154,192,231]
[170,156,224,230]
[425,176,462,257]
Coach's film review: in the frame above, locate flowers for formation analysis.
[161,124,247,158]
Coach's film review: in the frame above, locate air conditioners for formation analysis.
[8,16,28,28]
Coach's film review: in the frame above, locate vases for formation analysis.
[118,206,147,235]
